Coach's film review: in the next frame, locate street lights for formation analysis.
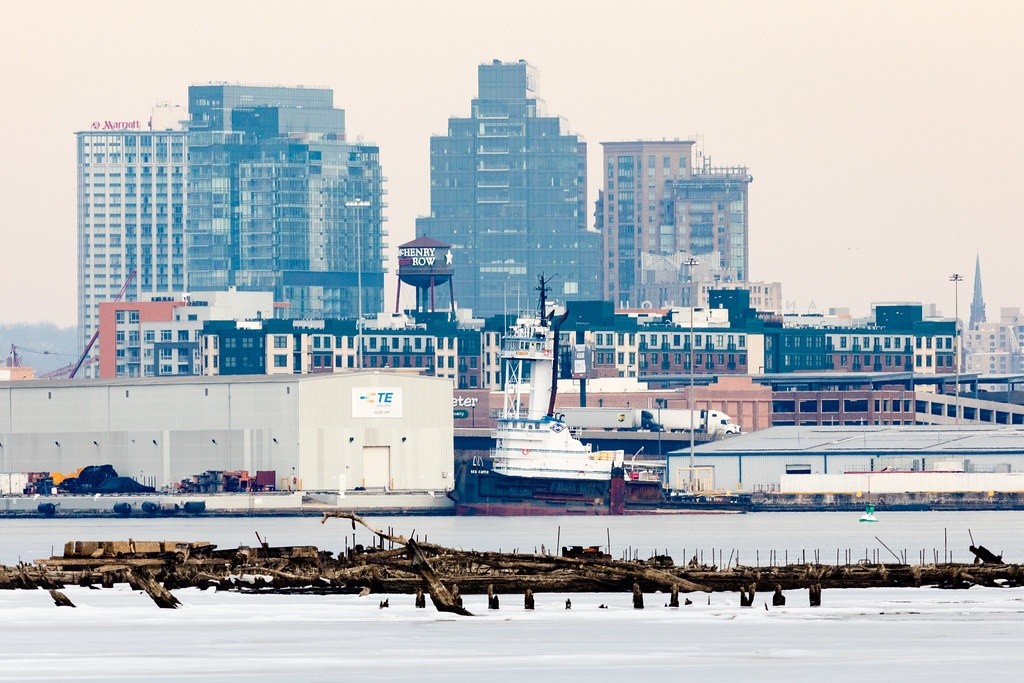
[682,256,700,488]
[345,198,371,372]
[949,273,964,424]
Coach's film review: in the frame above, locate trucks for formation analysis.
[554,407,666,432]
[644,409,741,434]
